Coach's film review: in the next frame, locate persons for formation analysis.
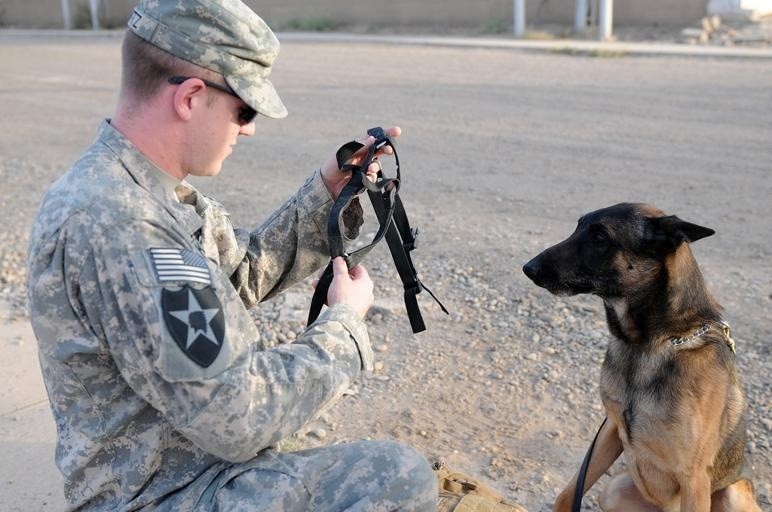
[27,0,440,511]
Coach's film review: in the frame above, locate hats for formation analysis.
[127,0,289,119]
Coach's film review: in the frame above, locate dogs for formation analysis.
[520,200,763,512]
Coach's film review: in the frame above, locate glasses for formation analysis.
[167,76,258,126]
